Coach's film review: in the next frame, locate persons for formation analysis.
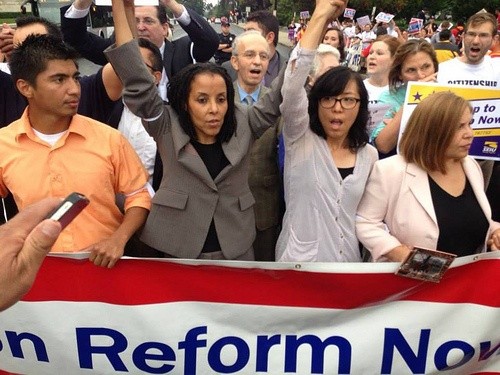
[0,0,500,264]
[0,34,152,269]
[0,197,64,311]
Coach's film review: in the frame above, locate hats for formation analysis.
[221,21,230,26]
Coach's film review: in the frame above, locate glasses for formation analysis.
[320,96,359,109]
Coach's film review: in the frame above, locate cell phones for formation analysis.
[48,192,91,232]
